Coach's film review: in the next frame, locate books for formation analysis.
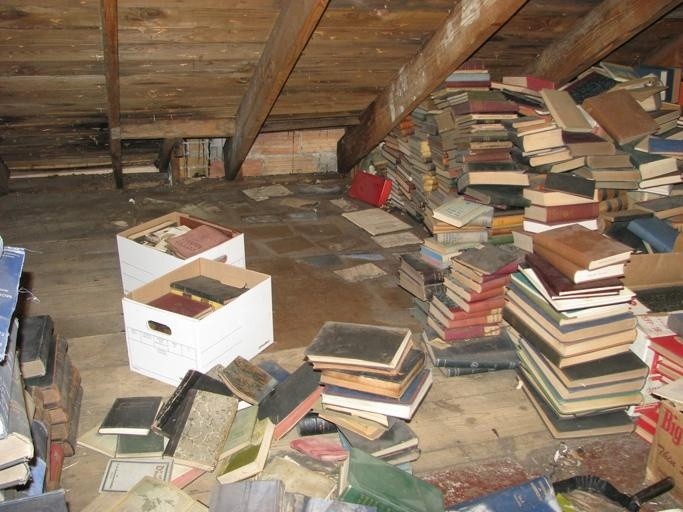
[0,237,81,512]
[304,321,431,422]
[502,224,649,439]
[126,215,231,260]
[95,356,444,510]
[142,274,250,334]
[626,286,682,418]
[635,312,683,443]
[372,64,683,252]
[422,243,531,377]
[397,238,486,328]
[444,476,561,512]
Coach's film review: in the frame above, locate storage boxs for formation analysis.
[646,400,682,506]
[122,255,275,387]
[116,210,247,296]
